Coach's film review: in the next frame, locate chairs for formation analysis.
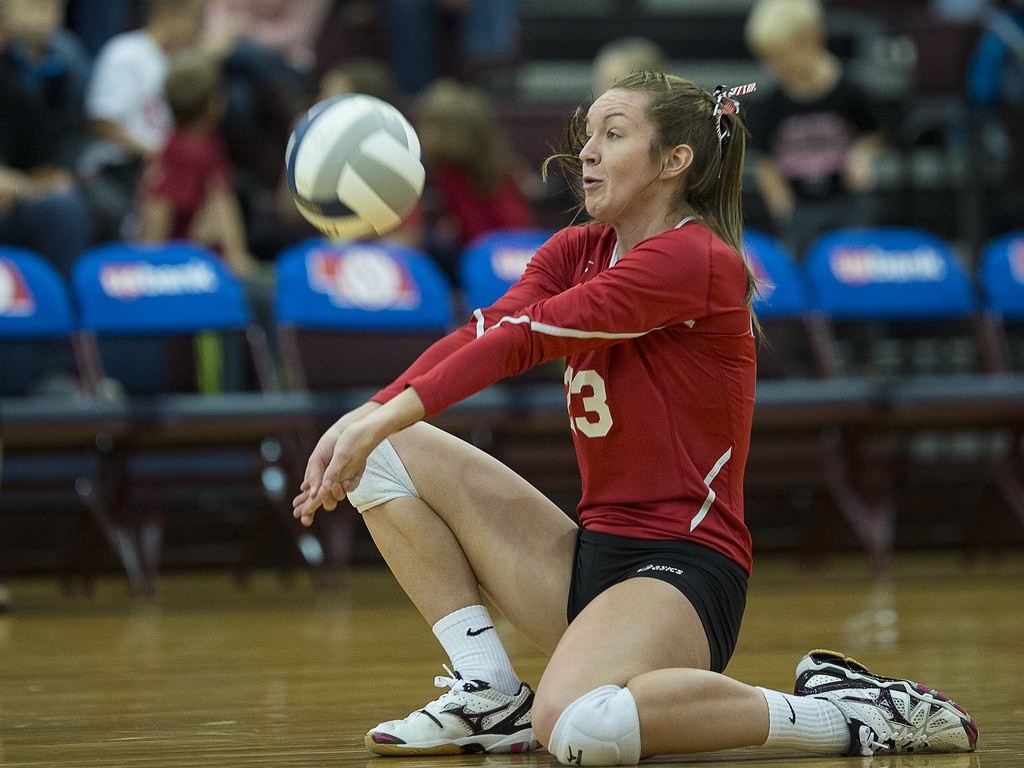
[0,228,1024,603]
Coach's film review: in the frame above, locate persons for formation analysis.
[0,0,1024,386]
[291,68,980,767]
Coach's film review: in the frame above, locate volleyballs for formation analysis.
[285,93,425,240]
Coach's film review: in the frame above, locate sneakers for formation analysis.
[365,663,543,755]
[792,649,979,755]
